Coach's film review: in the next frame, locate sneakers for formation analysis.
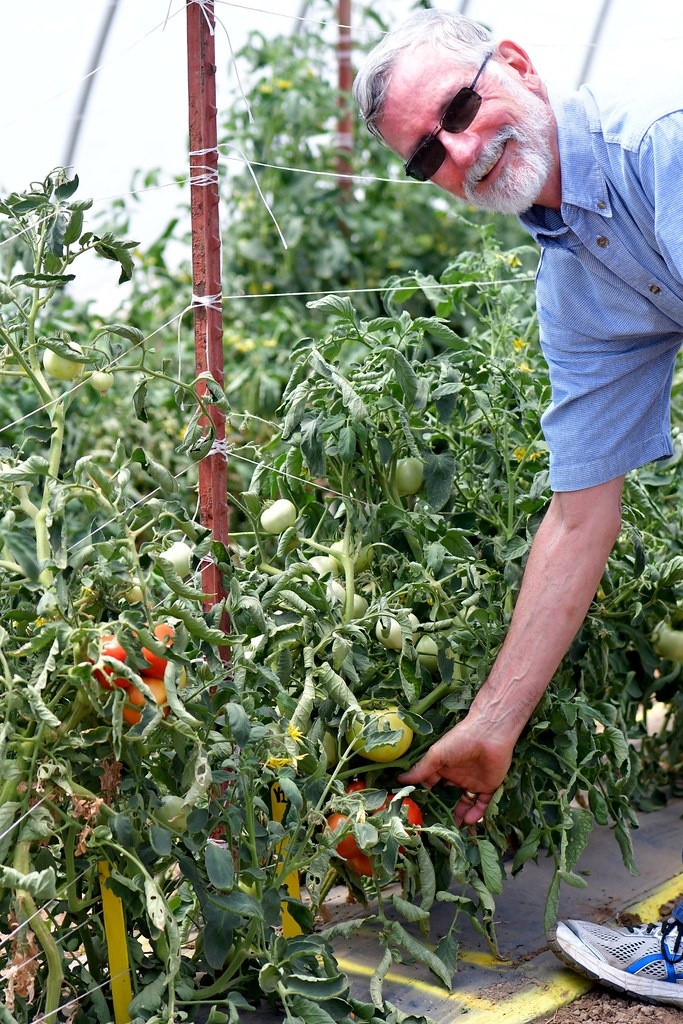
[548,902,683,1008]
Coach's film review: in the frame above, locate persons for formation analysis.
[350,8,683,1005]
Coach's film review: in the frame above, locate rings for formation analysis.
[462,789,478,800]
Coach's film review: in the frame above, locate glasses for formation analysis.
[403,53,498,183]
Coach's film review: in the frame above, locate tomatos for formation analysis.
[41,341,683,881]
